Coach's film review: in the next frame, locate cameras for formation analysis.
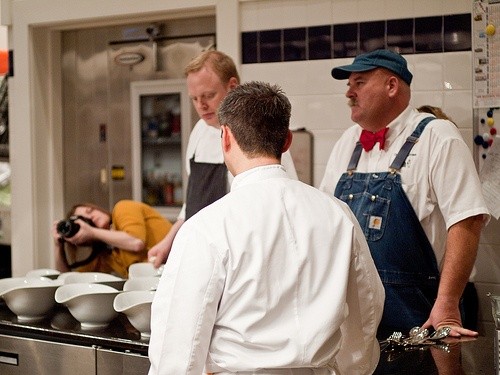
[56,216,95,238]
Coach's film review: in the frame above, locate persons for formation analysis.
[52,199,174,277]
[148,50,298,270]
[148,83,386,375]
[316,50,491,338]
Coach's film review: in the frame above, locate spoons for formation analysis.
[404,327,450,345]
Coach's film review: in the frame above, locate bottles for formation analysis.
[141,94,180,137]
[143,151,182,206]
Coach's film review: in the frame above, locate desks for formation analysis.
[375,326,496,375]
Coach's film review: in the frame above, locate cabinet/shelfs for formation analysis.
[130,78,200,223]
[0,300,151,375]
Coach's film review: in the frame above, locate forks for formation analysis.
[379,332,402,352]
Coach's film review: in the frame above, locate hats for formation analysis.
[331,50,413,87]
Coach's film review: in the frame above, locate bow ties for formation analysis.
[360,127,390,152]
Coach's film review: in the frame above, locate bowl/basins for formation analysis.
[113,291,157,337]
[57,272,127,290]
[26,269,61,279]
[0,277,62,320]
[123,263,166,291]
[55,284,121,327]
